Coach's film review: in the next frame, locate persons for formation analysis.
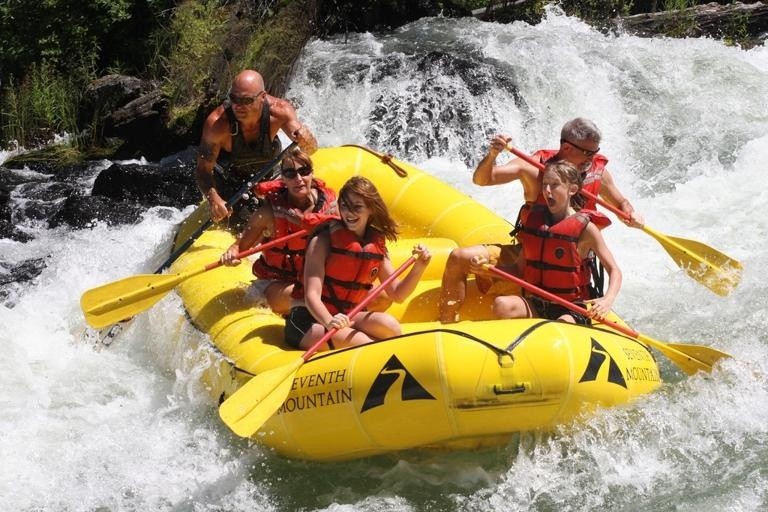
[219,150,338,315]
[198,68,319,223]
[439,116,645,322]
[287,177,432,351]
[470,161,622,335]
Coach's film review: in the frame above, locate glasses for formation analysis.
[229,91,265,105]
[563,138,600,156]
[282,165,312,178]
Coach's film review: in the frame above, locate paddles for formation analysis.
[506,141,741,295]
[218,255,421,439]
[83,132,300,348]
[80,228,311,329]
[478,254,735,377]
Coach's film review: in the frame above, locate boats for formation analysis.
[153,144,661,462]
